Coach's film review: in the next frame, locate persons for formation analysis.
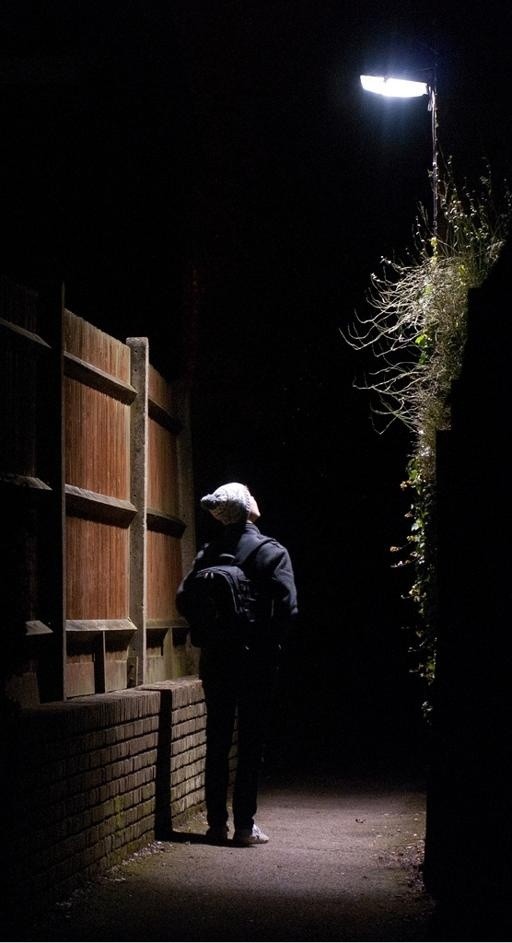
[173,481,301,847]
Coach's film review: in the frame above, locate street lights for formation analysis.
[357,40,447,272]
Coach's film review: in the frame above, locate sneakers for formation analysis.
[206,829,227,841]
[233,825,269,844]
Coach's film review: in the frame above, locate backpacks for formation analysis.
[188,532,275,647]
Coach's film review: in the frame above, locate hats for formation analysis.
[199,482,252,525]
